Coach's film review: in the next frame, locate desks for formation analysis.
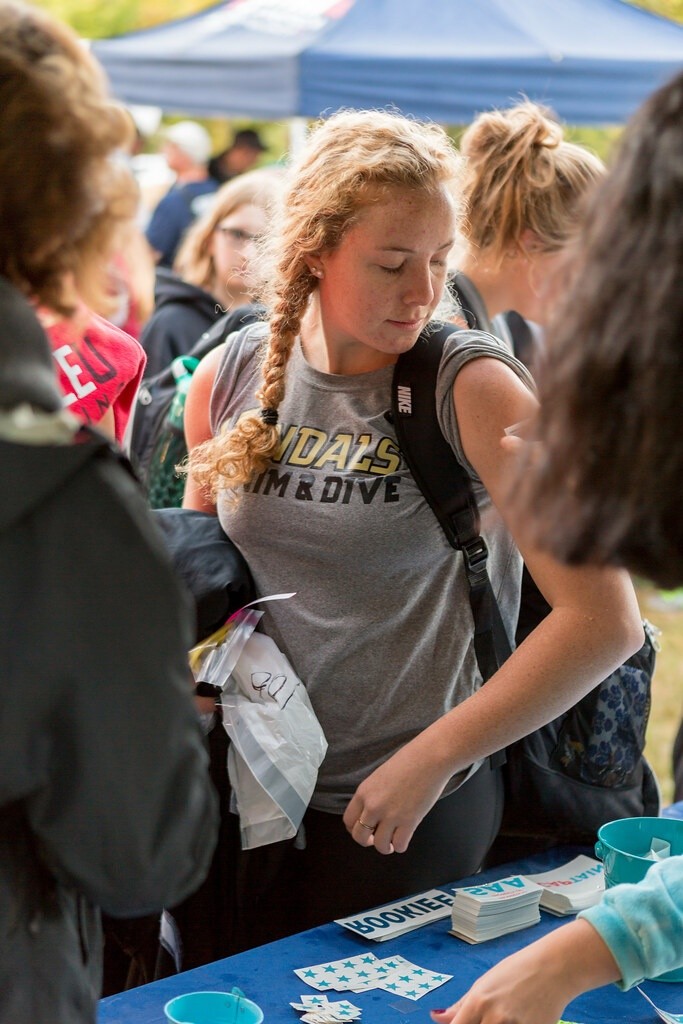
[95,800,683,1024]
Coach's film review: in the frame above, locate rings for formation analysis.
[356,819,377,830]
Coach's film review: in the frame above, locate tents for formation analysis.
[75,0,683,163]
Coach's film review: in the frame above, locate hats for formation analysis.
[159,120,212,165]
[235,130,267,151]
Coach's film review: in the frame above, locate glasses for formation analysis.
[214,226,265,250]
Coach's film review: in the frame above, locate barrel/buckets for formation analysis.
[594,817,683,982]
[164,991,264,1024]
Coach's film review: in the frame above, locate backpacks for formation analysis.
[388,322,658,846]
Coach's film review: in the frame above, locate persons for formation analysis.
[20,104,302,528]
[181,107,646,974]
[431,66,683,1024]
[1,0,222,1022]
